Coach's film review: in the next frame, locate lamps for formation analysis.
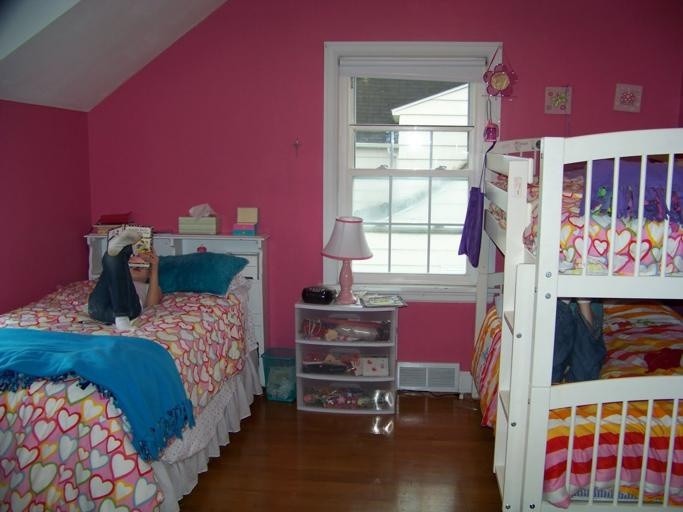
[320,215,373,305]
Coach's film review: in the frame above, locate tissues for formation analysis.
[176,203,221,235]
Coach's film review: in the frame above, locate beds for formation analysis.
[472,128,683,510]
[0,279,256,511]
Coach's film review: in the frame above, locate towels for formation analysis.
[457,186,485,269]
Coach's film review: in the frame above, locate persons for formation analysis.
[552,297,605,382]
[85,228,163,325]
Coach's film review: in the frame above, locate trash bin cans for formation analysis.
[261,348,296,407]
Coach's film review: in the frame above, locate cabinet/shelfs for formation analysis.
[295,298,399,415]
[82,230,271,391]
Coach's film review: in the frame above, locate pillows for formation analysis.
[150,252,249,294]
[225,274,246,298]
[579,156,682,225]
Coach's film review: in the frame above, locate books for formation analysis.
[105,223,154,270]
